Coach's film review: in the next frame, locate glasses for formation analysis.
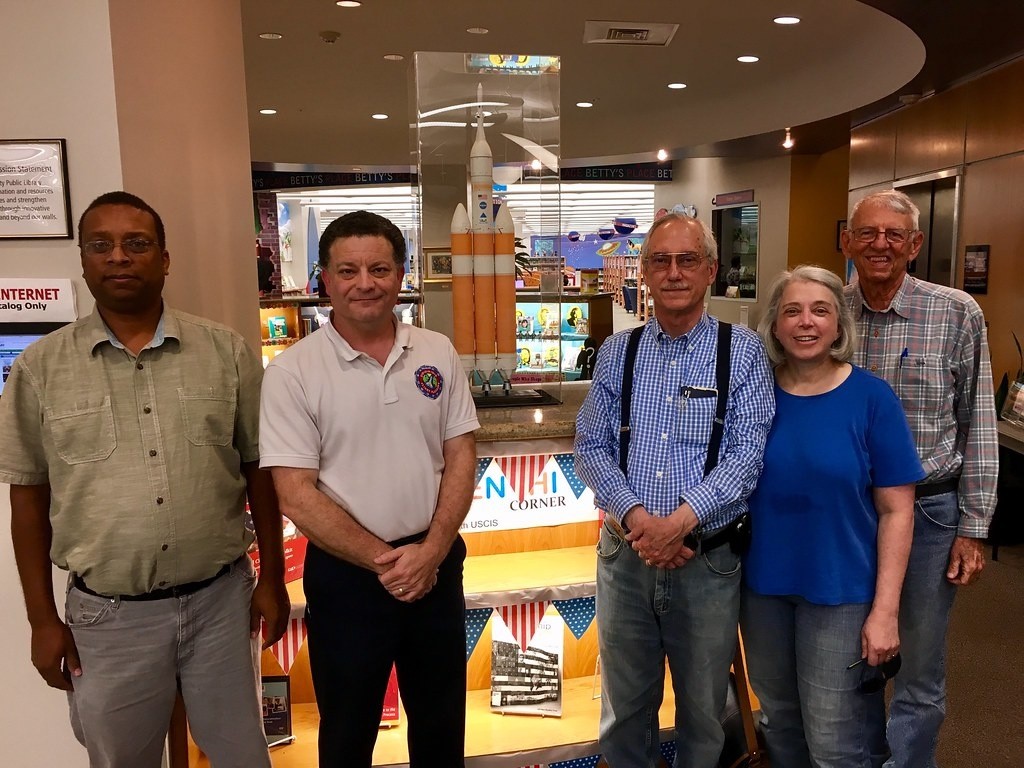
[849,653,902,695]
[850,226,916,243]
[82,237,166,254]
[645,251,710,271]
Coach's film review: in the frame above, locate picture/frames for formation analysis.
[837,219,847,253]
[0,139,74,242]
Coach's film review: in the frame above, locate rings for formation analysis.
[398,587,404,596]
[887,655,893,658]
[648,559,652,566]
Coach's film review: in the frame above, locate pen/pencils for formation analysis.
[900,348,908,370]
[847,658,867,670]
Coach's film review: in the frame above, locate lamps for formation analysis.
[781,127,793,148]
[321,34,336,45]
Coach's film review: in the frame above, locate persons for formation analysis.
[259,209,480,768]
[1,191,291,768]
[572,211,776,768]
[731,265,925,768]
[832,188,1000,768]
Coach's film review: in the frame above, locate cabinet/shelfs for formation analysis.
[515,254,655,325]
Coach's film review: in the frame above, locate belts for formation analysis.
[605,513,729,554]
[73,554,244,601]
[915,478,959,500]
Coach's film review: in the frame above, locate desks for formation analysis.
[992,419,1024,562]
